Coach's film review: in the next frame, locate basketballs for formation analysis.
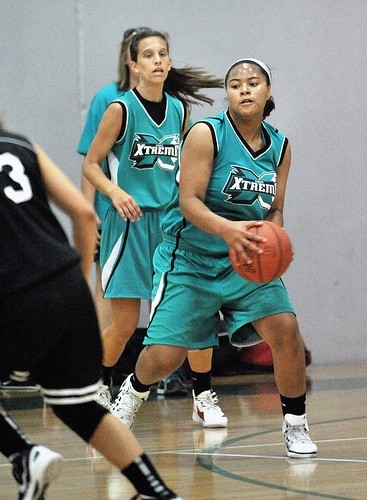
[231,219,295,282]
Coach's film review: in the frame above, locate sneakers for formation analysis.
[282,413,317,458]
[18,444,64,500]
[95,385,112,409]
[109,373,151,431]
[192,388,228,428]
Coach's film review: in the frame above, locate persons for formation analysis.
[109,58,317,457]
[78,29,151,333]
[90,27,229,429]
[0,111,179,500]
